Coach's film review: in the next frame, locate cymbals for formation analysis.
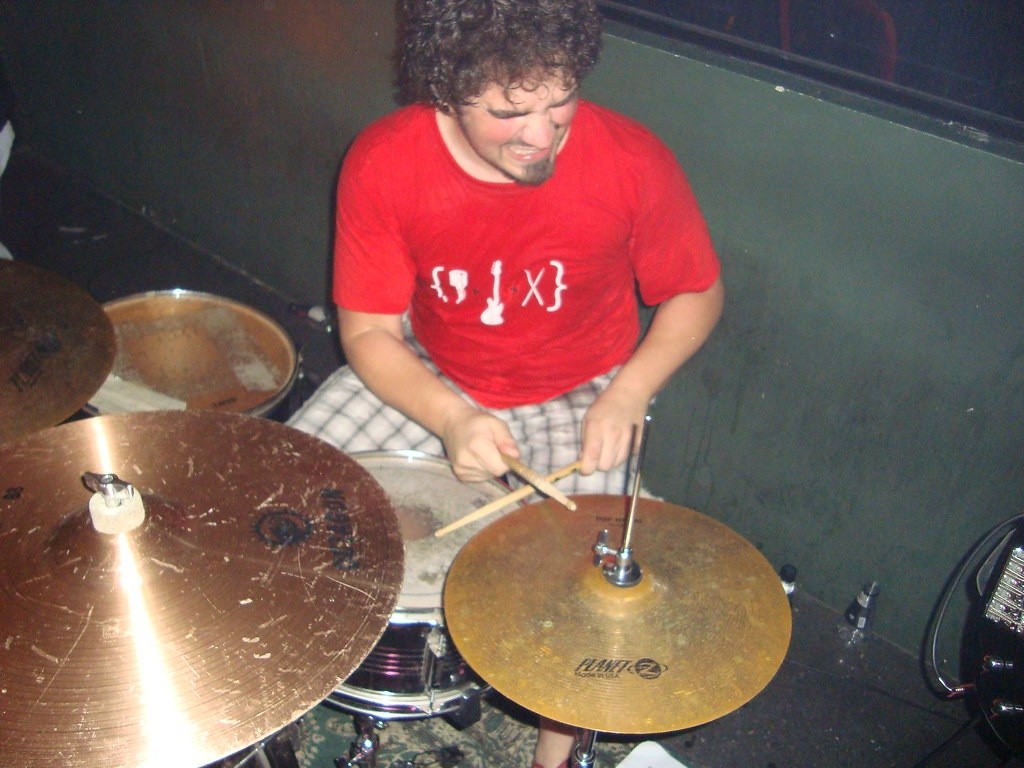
[0,258,119,431]
[438,491,796,736]
[0,407,405,768]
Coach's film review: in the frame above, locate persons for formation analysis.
[280,0,723,768]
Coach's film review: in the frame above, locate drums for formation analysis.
[319,451,528,720]
[67,287,302,431]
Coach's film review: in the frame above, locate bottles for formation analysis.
[833,580,882,648]
[778,565,798,606]
